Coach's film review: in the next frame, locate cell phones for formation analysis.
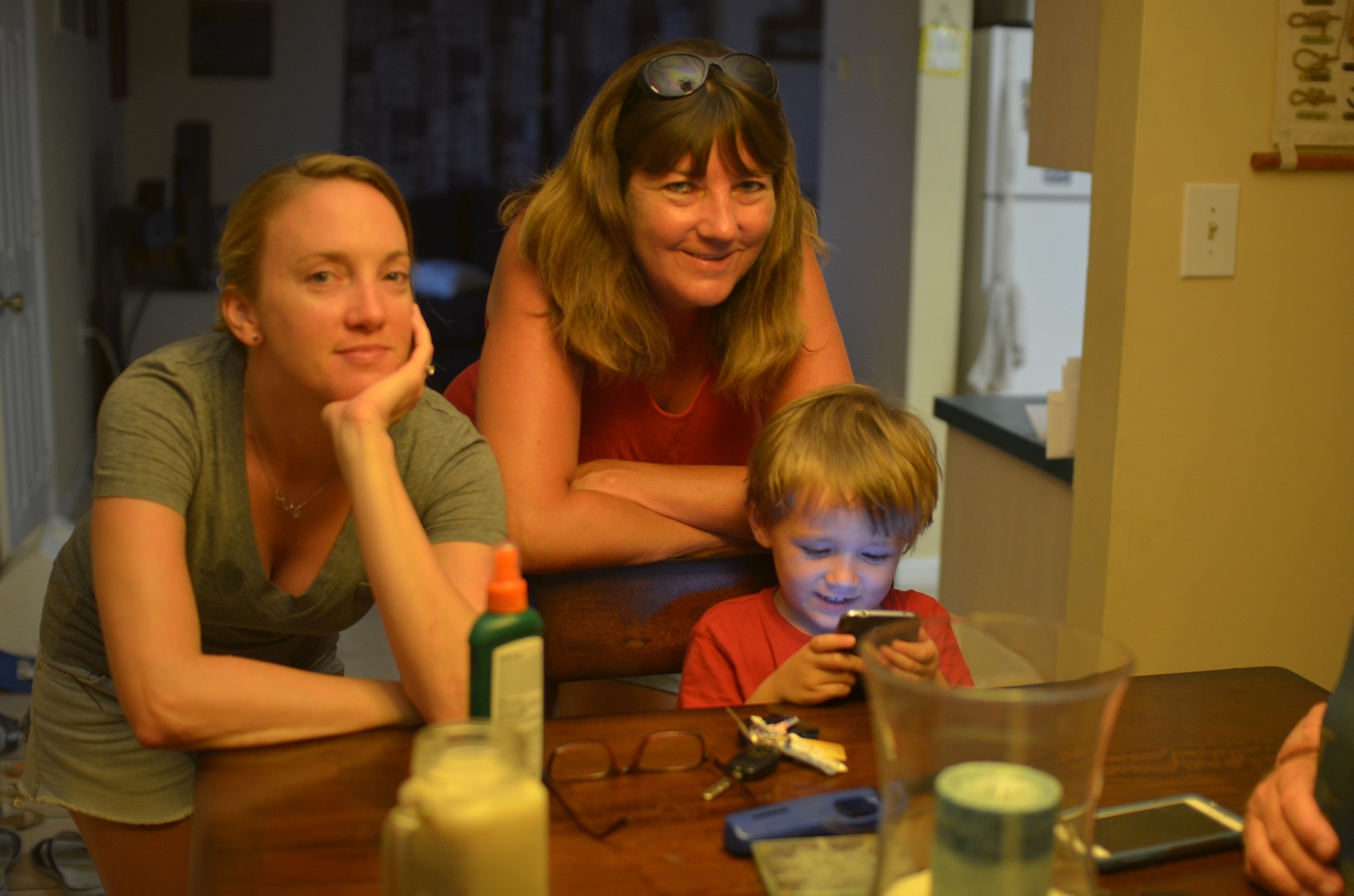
[835,606,923,659]
[1052,791,1251,875]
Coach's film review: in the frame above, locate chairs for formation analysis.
[521,553,778,718]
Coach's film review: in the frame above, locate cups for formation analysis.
[855,610,1138,896]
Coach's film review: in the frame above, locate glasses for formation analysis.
[542,729,764,840]
[627,54,779,103]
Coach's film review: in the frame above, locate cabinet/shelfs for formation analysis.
[935,396,1073,664]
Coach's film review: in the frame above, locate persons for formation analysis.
[676,382,975,709]
[1243,622,1354,896]
[442,38,857,573]
[15,154,509,895]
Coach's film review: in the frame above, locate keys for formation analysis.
[704,714,848,802]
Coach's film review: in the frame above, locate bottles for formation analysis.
[375,715,550,896]
[927,759,1065,896]
[468,541,548,787]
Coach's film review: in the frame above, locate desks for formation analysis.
[1,667,1338,896]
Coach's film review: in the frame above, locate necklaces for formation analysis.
[243,389,341,517]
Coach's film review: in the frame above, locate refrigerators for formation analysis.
[953,28,1092,400]
